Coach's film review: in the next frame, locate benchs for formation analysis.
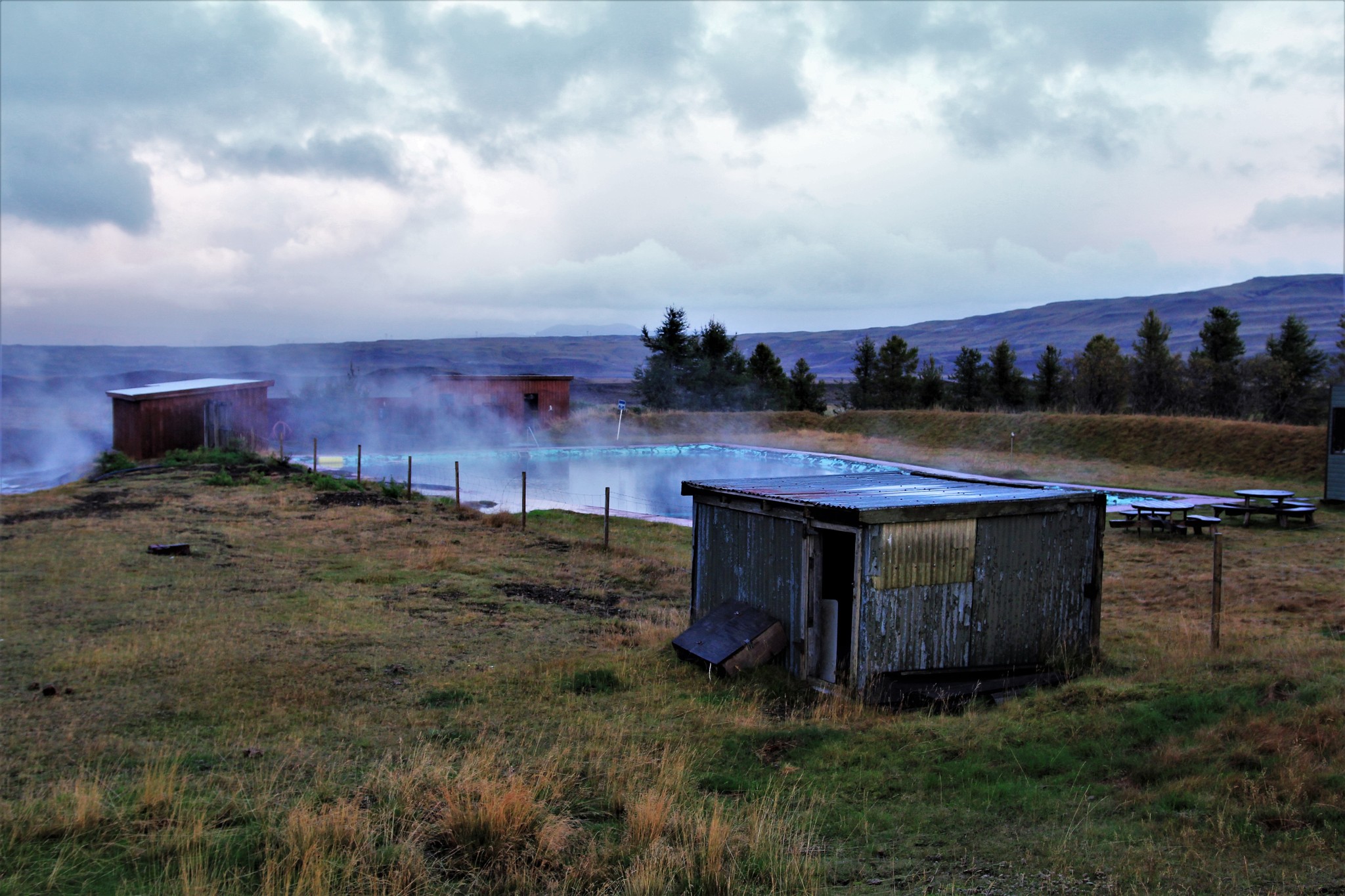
[1210,501,1251,522]
[1263,498,1317,509]
[1115,509,1172,536]
[1279,507,1319,529]
[1184,512,1223,538]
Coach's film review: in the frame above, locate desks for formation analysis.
[1233,488,1298,529]
[1130,499,1197,537]
